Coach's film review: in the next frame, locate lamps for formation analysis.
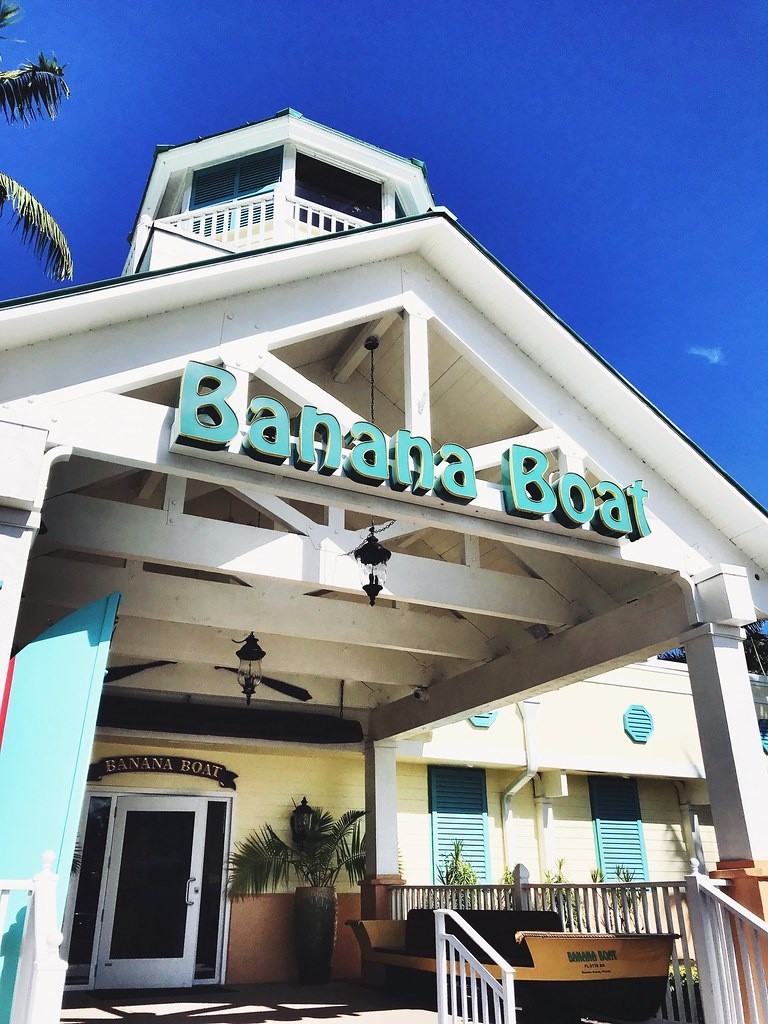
[353,335,392,610]
[236,631,266,706]
[291,796,316,852]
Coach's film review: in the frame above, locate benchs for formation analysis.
[344,908,684,1024]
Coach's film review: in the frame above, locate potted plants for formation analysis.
[222,808,404,986]
